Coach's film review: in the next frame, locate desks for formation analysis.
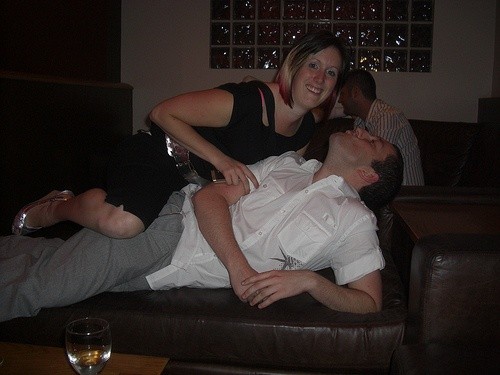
[391,203,500,242]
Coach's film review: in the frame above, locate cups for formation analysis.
[65,318,111,375]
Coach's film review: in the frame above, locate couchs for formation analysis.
[0,202,409,375]
[389,233,500,375]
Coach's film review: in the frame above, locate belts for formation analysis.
[172,140,209,186]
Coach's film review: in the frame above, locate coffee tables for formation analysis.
[0,343,170,375]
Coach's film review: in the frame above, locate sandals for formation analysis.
[12,189,75,236]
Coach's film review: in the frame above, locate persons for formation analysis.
[0,127,404,322]
[12,30,346,239]
[338,65,425,186]
